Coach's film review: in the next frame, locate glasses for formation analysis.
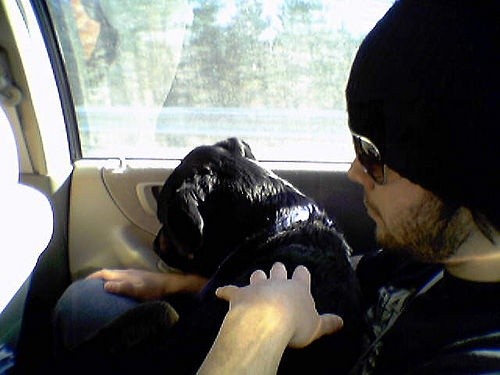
[350,134,385,185]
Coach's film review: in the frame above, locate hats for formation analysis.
[346,0,500,214]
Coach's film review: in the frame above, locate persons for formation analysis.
[44,1,500,375]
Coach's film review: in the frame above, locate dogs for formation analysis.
[54,137,367,375]
